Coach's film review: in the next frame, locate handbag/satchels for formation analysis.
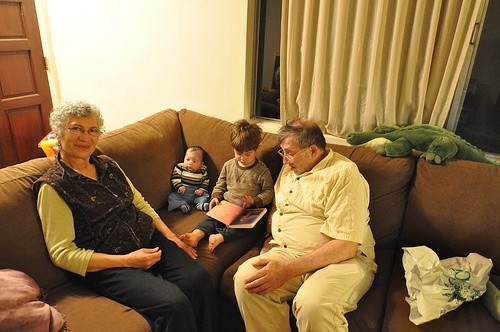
[402,245,493,325]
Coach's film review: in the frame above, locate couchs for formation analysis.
[0,109,500,332]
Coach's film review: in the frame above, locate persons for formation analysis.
[32,98,223,332]
[167,146,211,212]
[178,119,274,252]
[232,117,377,332]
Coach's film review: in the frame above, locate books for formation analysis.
[206,200,267,228]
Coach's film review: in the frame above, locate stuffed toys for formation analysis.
[345,121,500,164]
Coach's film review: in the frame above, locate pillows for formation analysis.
[0,267,69,332]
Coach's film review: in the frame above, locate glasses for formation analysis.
[66,125,103,138]
[277,147,309,164]
